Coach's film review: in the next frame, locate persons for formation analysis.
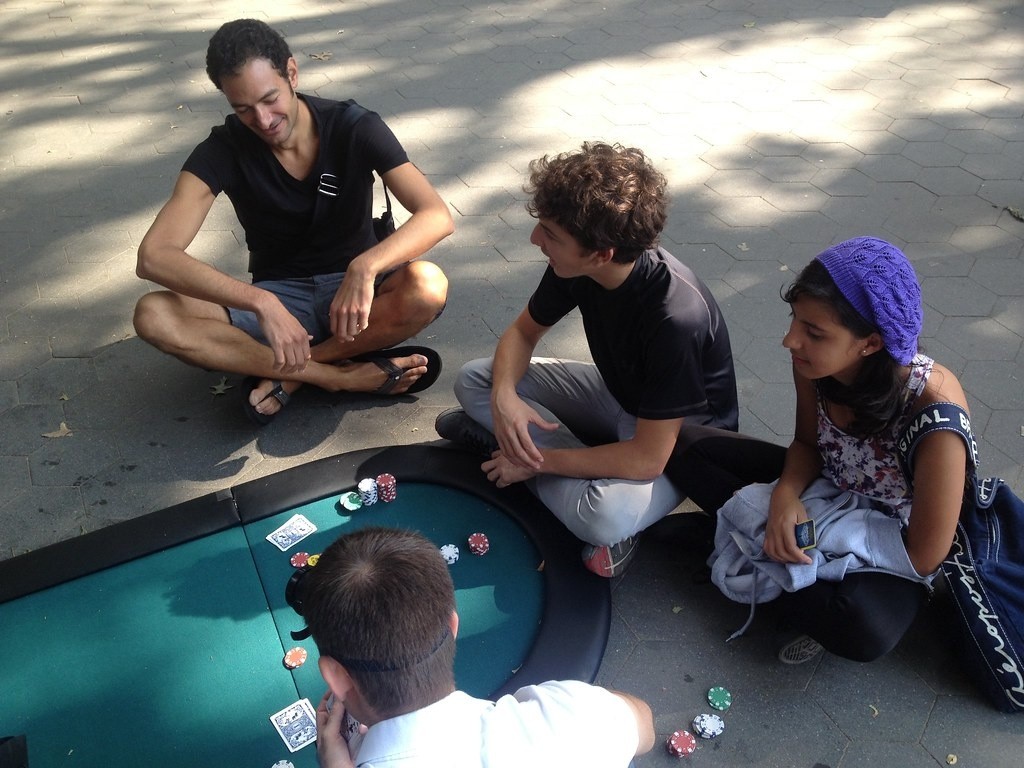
[302,527,657,768]
[663,237,970,664]
[132,19,455,424]
[435,140,740,578]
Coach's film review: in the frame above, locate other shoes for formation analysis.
[580,530,643,577]
[778,633,824,664]
[435,406,500,457]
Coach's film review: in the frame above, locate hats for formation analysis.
[815,236,922,366]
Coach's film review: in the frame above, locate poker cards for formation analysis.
[325,691,365,764]
[269,697,317,754]
[265,513,317,553]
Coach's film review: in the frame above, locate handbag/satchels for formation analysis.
[897,401,1024,715]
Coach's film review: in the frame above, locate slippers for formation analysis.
[236,375,292,426]
[334,346,442,396]
